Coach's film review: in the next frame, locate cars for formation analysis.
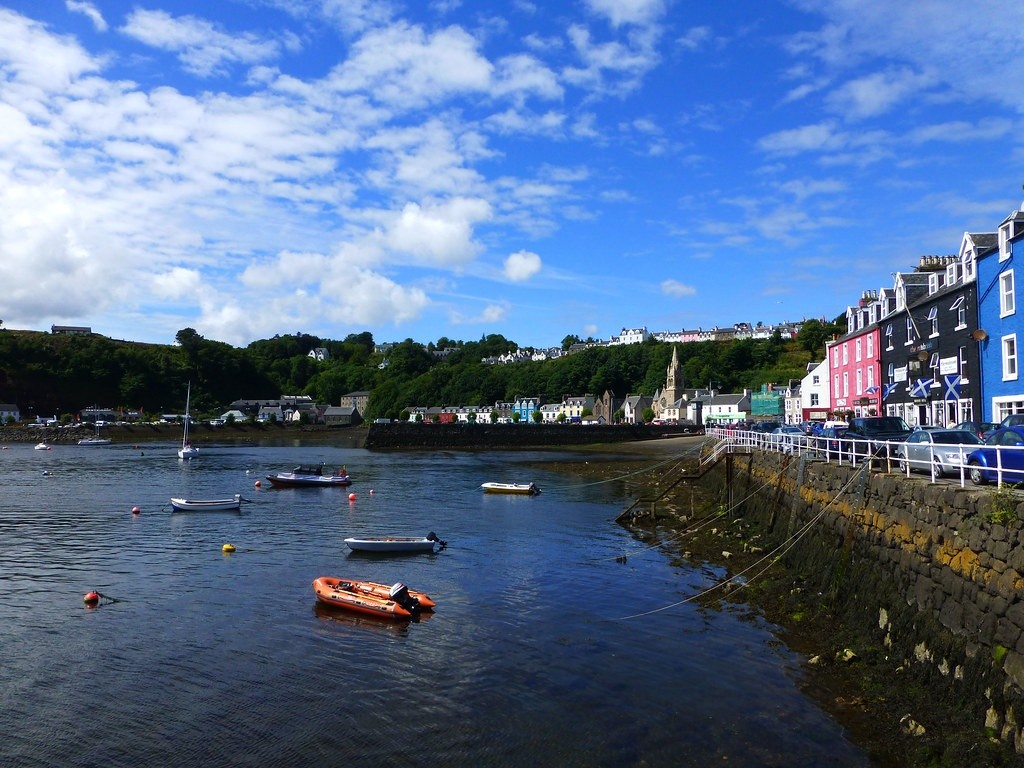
[980,413,1024,440]
[952,420,1007,445]
[713,419,849,458]
[910,425,944,435]
[967,425,1024,484]
[897,428,985,479]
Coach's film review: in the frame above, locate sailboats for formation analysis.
[78,403,112,445]
[177,380,200,458]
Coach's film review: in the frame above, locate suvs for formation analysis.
[844,415,914,467]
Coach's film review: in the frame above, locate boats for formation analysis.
[311,577,437,619]
[35,443,49,450]
[264,464,352,486]
[170,494,251,511]
[480,481,542,495]
[344,532,448,553]
